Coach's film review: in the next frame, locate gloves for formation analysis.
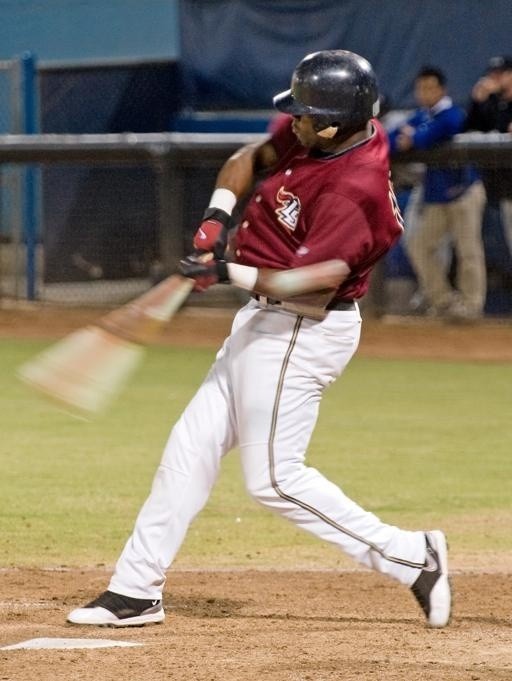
[177,249,230,292]
[193,207,232,259]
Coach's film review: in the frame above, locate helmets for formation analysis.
[272,49,379,139]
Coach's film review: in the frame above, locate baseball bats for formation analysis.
[29,252,214,410]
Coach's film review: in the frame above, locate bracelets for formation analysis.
[208,188,238,215]
[226,263,258,292]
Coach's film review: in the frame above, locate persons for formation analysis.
[462,53,512,255]
[386,65,488,323]
[66,48,452,630]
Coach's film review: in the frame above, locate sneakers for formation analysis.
[68,590,165,626]
[409,531,451,628]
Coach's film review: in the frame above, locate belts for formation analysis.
[255,293,356,310]
